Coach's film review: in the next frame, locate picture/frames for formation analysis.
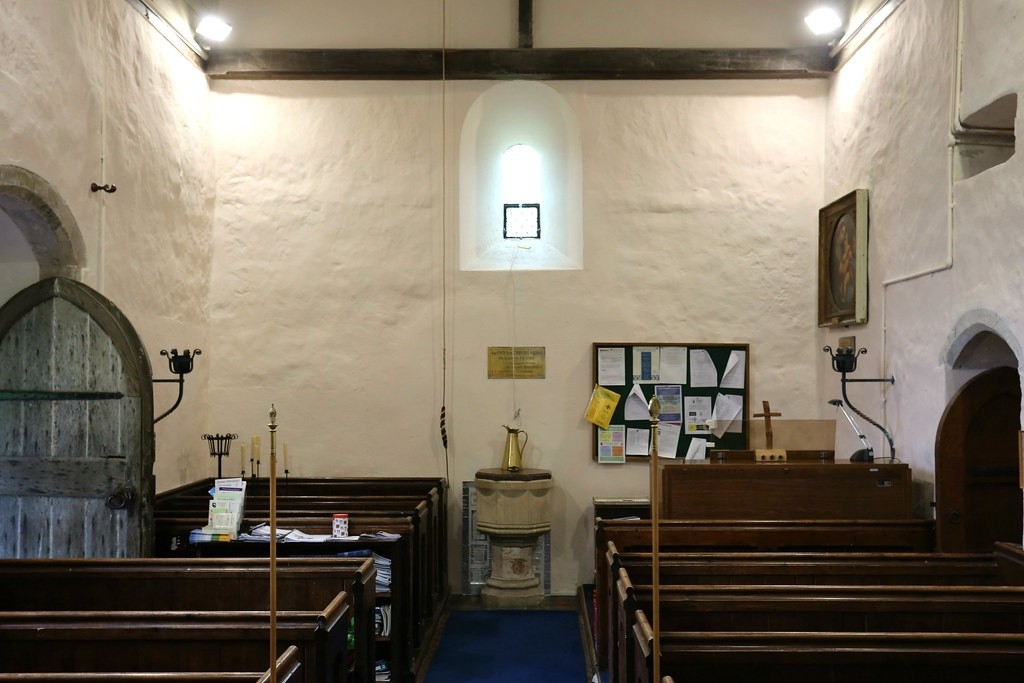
[816,189,869,329]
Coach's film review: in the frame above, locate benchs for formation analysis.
[593,516,1024,683]
[0,475,450,683]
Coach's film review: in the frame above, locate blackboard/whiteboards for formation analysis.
[592,342,750,460]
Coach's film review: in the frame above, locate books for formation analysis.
[376,671,392,682]
[381,605,391,636]
[209,477,247,540]
[371,552,391,593]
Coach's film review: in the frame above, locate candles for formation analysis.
[283,442,288,470]
[241,444,246,470]
[250,435,261,460]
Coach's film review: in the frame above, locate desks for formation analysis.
[474,467,552,535]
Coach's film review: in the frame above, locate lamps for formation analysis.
[804,6,845,40]
[194,13,230,44]
[827,399,874,462]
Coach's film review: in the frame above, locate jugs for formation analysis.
[500,424,528,472]
